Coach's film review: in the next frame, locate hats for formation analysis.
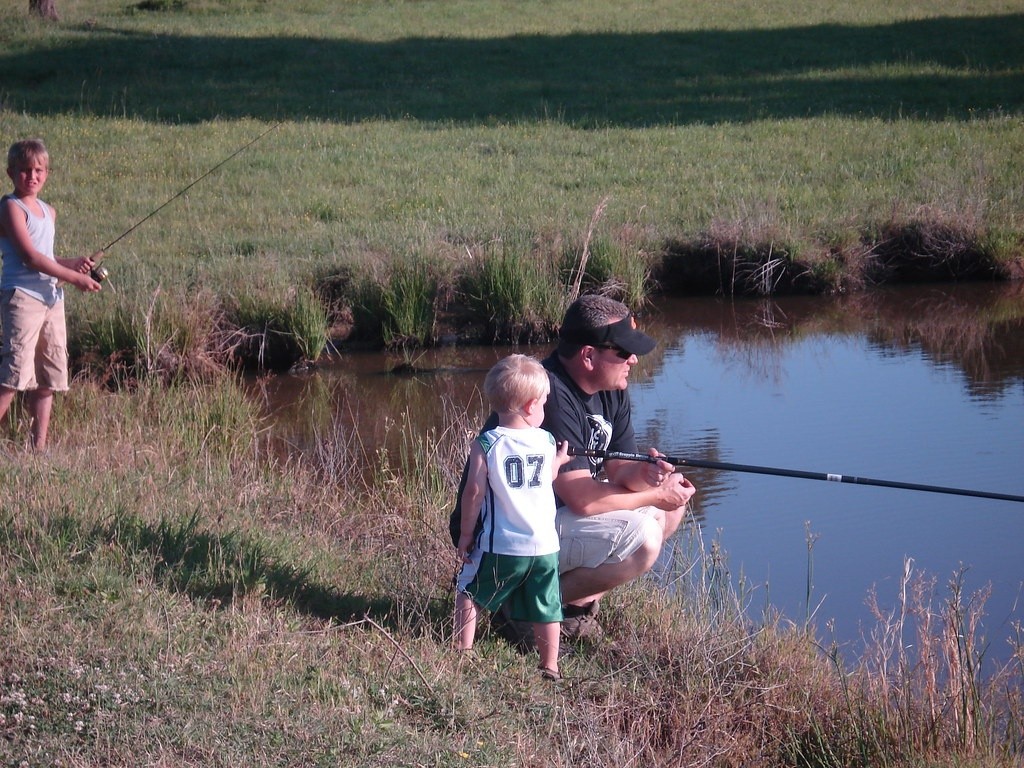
[559,309,657,355]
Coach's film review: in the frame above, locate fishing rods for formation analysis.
[77,67,409,289]
[556,442,1023,503]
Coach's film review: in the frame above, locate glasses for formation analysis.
[577,343,632,359]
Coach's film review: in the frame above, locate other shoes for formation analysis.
[560,600,621,655]
[489,600,567,657]
[453,646,478,661]
[537,666,565,684]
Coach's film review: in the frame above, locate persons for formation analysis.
[447,294,698,645]
[0,139,103,451]
[451,354,577,683]
